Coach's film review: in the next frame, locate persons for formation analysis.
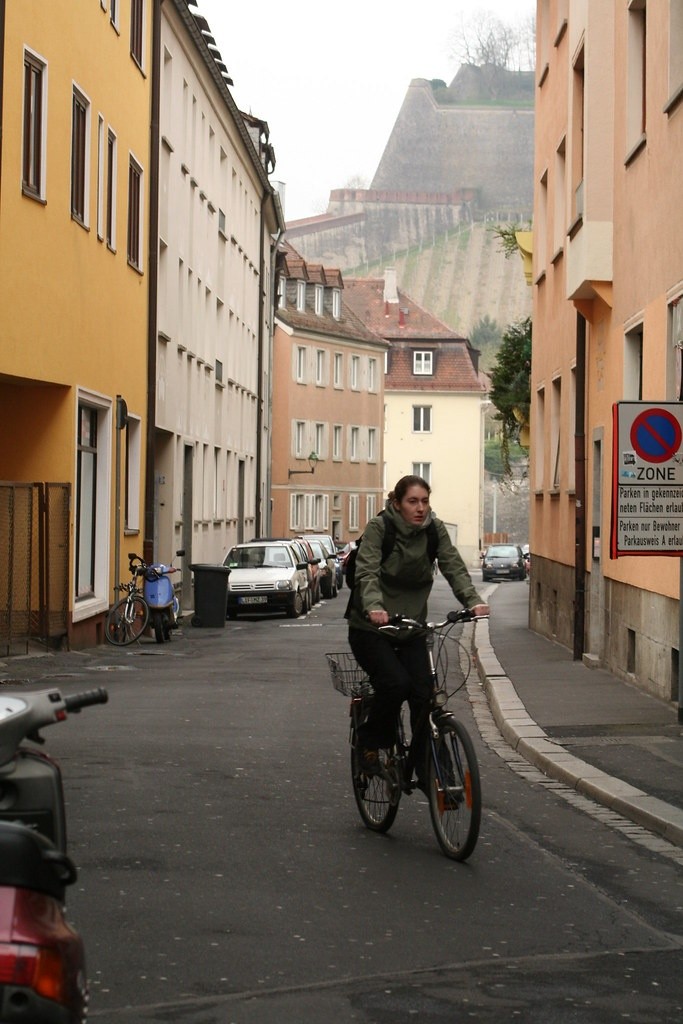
[344,474,490,804]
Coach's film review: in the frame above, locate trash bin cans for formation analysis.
[187,563,232,629]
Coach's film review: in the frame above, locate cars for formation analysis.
[220,540,308,620]
[270,532,359,612]
[479,543,529,582]
[519,544,530,572]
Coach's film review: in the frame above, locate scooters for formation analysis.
[0,678,110,1023]
[128,549,186,645]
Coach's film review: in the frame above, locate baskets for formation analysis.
[325,651,375,698]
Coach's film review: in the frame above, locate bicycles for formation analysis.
[326,607,490,863]
[104,564,160,647]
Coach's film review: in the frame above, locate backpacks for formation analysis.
[343,510,439,620]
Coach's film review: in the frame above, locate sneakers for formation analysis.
[355,740,382,776]
[445,783,463,807]
